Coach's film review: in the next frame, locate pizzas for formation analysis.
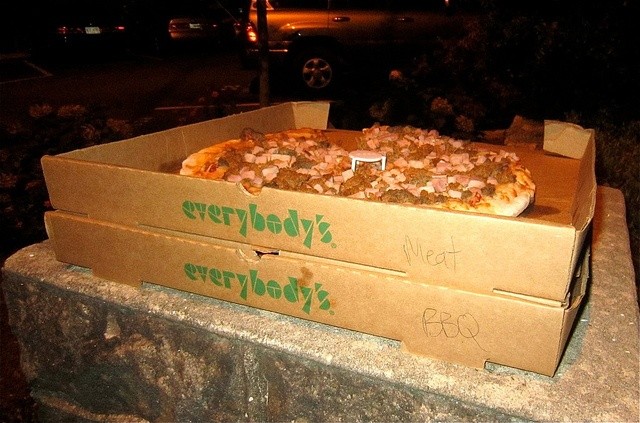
[179,123,537,217]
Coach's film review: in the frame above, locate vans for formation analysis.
[168,7,221,41]
[244,0,449,87]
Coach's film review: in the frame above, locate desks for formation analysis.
[1,188,639,421]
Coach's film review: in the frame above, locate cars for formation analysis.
[52,4,135,53]
